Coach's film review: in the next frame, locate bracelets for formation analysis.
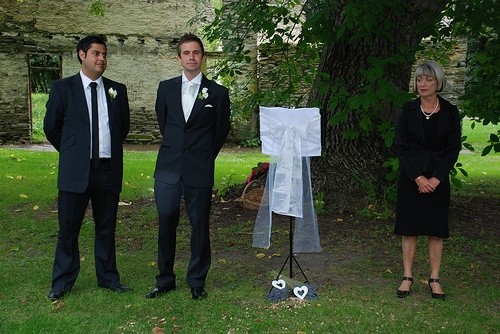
[415,176,421,181]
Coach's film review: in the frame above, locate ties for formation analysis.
[90,82,99,165]
[183,82,195,122]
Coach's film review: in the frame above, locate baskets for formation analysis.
[233,179,265,214]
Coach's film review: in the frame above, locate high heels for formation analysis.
[396,277,413,298]
[429,278,445,301]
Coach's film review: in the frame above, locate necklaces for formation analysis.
[420,97,440,119]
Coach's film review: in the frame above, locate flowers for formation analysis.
[109,87,117,103]
[197,87,209,100]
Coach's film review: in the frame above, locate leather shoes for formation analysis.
[109,284,133,293]
[48,291,64,300]
[146,283,176,298]
[191,287,207,300]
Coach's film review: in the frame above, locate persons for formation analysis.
[393,61,462,299]
[146,36,231,299]
[47,36,135,300]
